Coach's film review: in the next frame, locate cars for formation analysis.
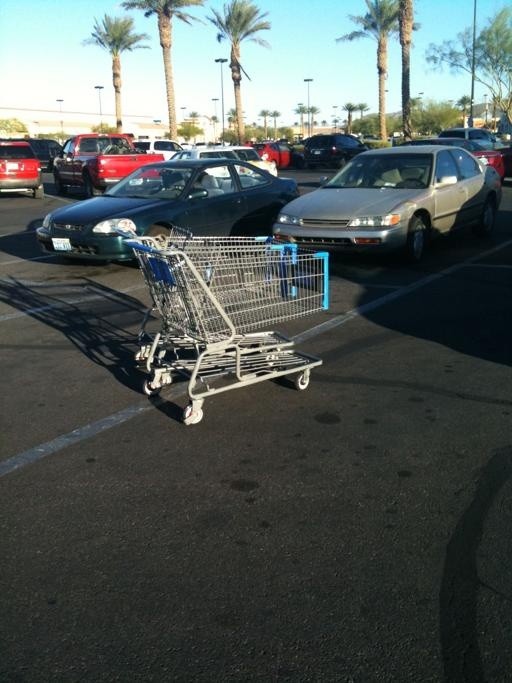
[35,159,299,264]
[400,137,506,180]
[0,140,44,198]
[271,145,501,261]
[438,127,505,150]
[133,128,369,167]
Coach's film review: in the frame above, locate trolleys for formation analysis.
[118,229,329,425]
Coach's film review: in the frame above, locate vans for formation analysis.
[19,137,64,173]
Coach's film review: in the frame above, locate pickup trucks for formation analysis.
[52,133,165,197]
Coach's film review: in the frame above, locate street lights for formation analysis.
[303,78,313,136]
[215,57,228,145]
